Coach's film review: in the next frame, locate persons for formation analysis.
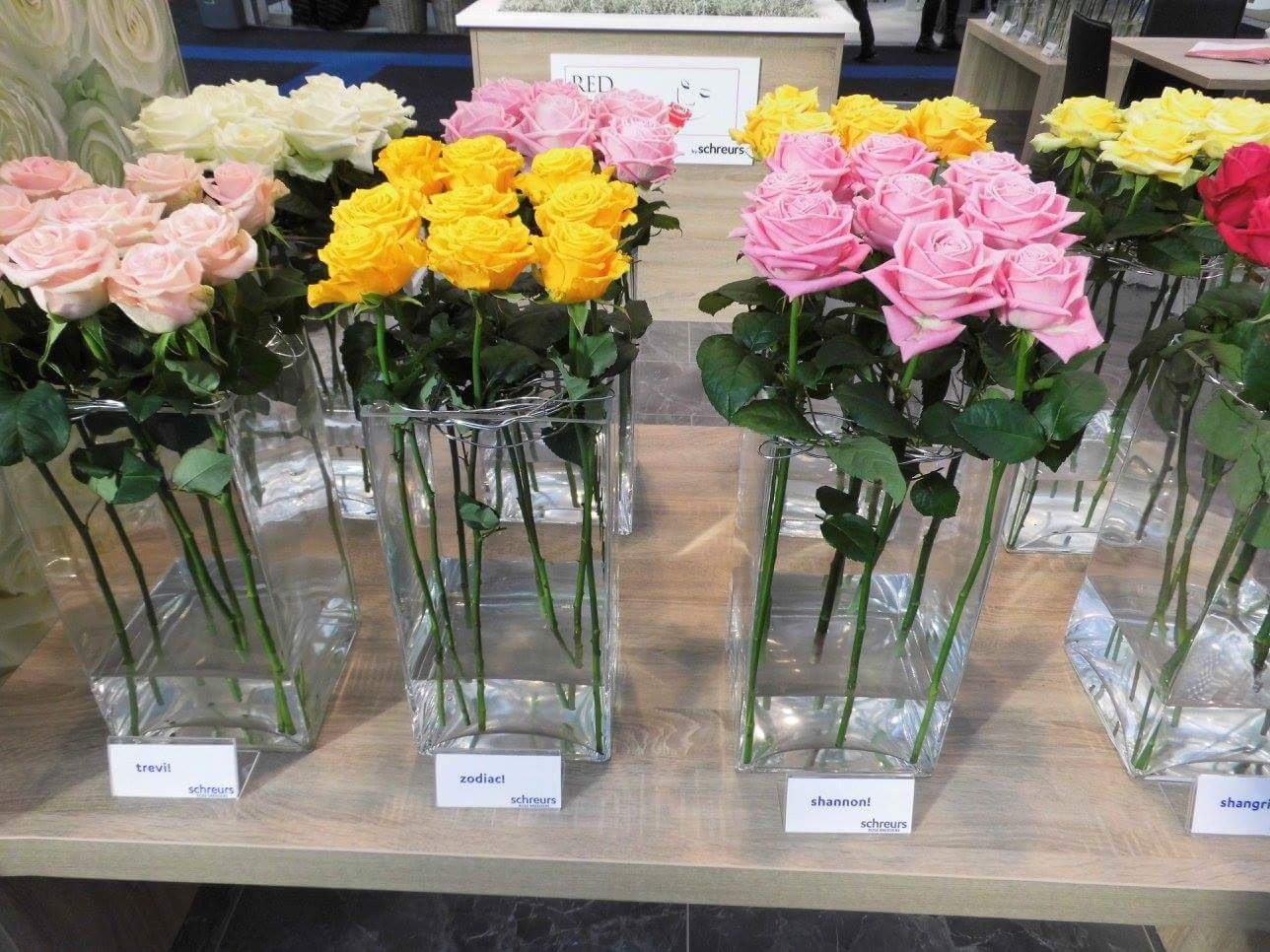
[914,0,962,53]
[846,0,879,60]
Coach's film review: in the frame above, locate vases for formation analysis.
[0,332,357,754]
[359,392,613,761]
[478,245,637,534]
[999,249,1181,556]
[1064,336,1270,778]
[727,396,1018,780]
[226,321,435,527]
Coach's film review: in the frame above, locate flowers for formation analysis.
[0,72,1270,776]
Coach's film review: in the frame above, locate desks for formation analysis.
[1112,37,1270,97]
[0,424,1270,952]
[956,19,1131,163]
